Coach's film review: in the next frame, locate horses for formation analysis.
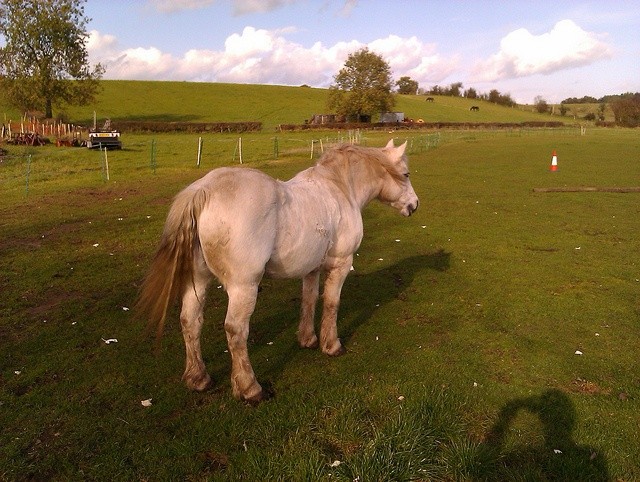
[470,106,479,112]
[426,97,434,102]
[131,138,420,407]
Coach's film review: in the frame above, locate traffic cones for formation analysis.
[550,150,558,170]
